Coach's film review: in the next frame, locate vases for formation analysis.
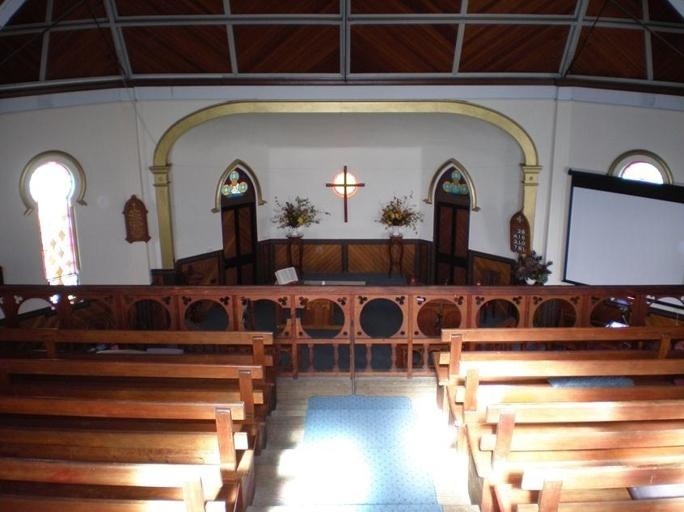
[391,225,400,237]
[524,276,536,286]
[288,227,303,238]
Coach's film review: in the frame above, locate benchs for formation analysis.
[431,325,684,512]
[1,327,277,512]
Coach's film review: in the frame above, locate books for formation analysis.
[275,266,298,286]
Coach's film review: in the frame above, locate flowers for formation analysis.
[515,251,553,283]
[270,194,332,235]
[373,190,426,236]
